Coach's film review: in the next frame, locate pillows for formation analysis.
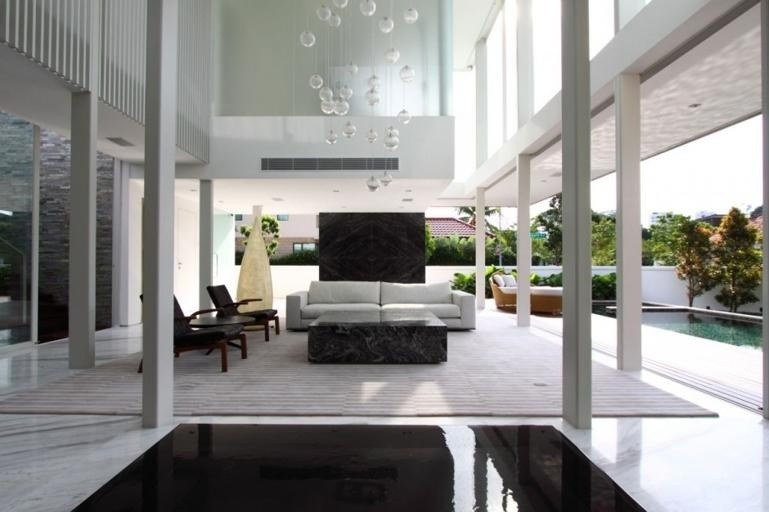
[503,275,516,287]
[494,274,505,287]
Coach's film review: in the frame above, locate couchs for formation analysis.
[286,280,477,331]
[488,271,563,316]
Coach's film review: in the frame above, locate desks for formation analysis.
[189,317,256,355]
[72,423,456,510]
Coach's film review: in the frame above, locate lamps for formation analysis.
[300,0,419,192]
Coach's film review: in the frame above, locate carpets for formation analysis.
[0,317,719,418]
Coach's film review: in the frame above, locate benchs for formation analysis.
[468,422,648,511]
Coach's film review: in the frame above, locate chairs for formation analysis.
[206,284,279,341]
[138,293,248,373]
